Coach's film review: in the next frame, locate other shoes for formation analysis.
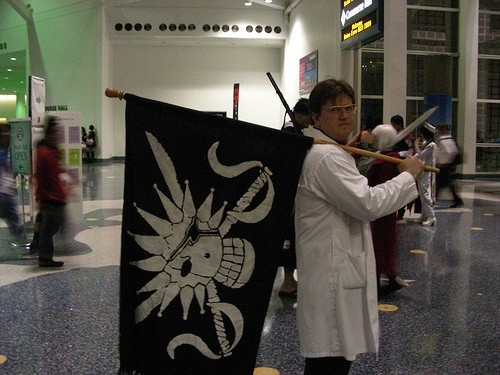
[448,199,464,208]
[19,250,40,259]
[382,281,402,295]
[39,260,64,267]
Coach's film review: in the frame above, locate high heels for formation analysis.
[415,214,437,226]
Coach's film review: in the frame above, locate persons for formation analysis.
[281,97,304,294]
[391,115,415,155]
[415,127,437,227]
[363,124,406,289]
[87,125,95,162]
[0,122,24,246]
[81,127,87,158]
[30,114,65,267]
[435,125,463,208]
[361,127,373,157]
[293,78,426,374]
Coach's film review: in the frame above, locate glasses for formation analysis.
[318,104,357,116]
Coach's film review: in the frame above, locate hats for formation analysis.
[423,121,436,133]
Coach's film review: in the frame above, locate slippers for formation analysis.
[278,281,298,299]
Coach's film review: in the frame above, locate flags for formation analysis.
[116,94,315,375]
